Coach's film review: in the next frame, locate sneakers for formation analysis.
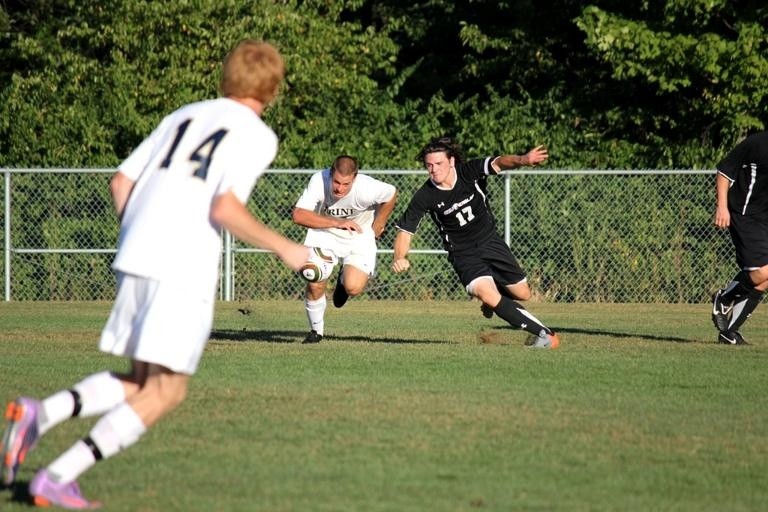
[480,302,494,319]
[332,264,350,309]
[302,329,323,344]
[718,330,750,346]
[28,466,102,511]
[523,329,560,350]
[0,396,43,488]
[711,288,735,332]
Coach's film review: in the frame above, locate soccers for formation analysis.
[301,246,337,284]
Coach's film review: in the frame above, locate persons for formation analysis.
[711,129,768,346]
[391,136,560,351]
[292,156,397,344]
[1,38,335,510]
